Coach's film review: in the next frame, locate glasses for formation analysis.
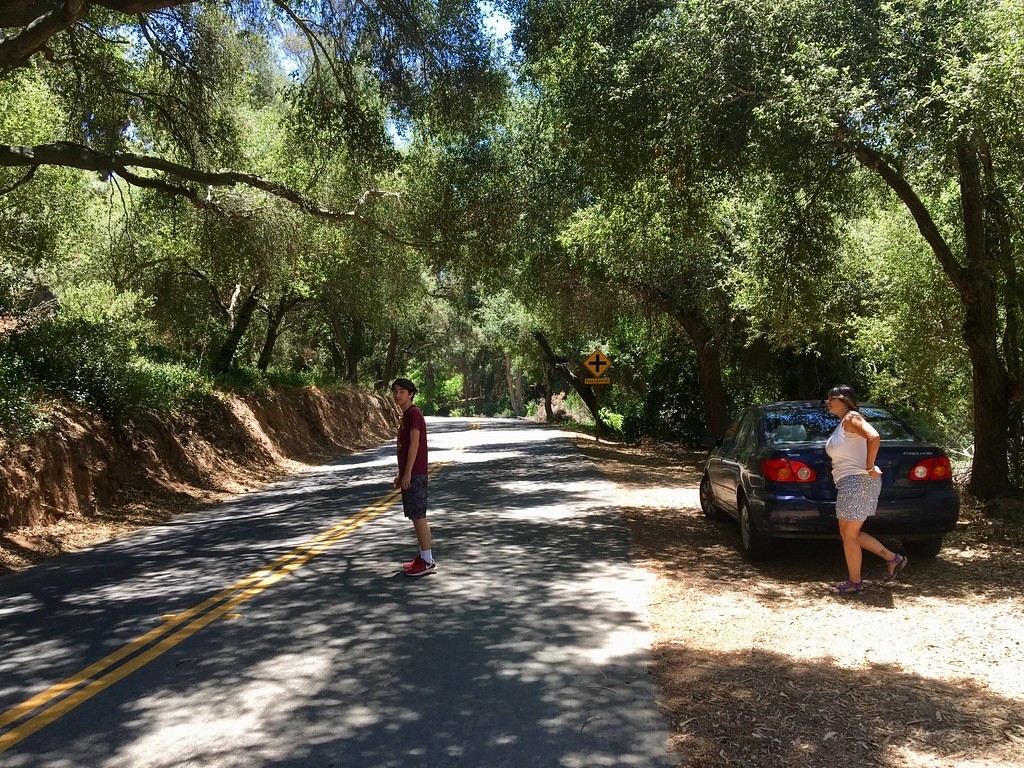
[828,395,841,400]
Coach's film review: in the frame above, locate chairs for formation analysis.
[773,423,808,441]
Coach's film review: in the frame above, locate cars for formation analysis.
[699,400,961,567]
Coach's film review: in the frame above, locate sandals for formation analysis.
[883,553,908,582]
[830,580,867,596]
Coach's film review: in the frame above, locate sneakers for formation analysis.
[403,555,438,576]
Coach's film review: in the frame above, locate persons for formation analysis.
[825,384,908,595]
[391,377,439,577]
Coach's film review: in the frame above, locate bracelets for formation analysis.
[866,465,875,473]
